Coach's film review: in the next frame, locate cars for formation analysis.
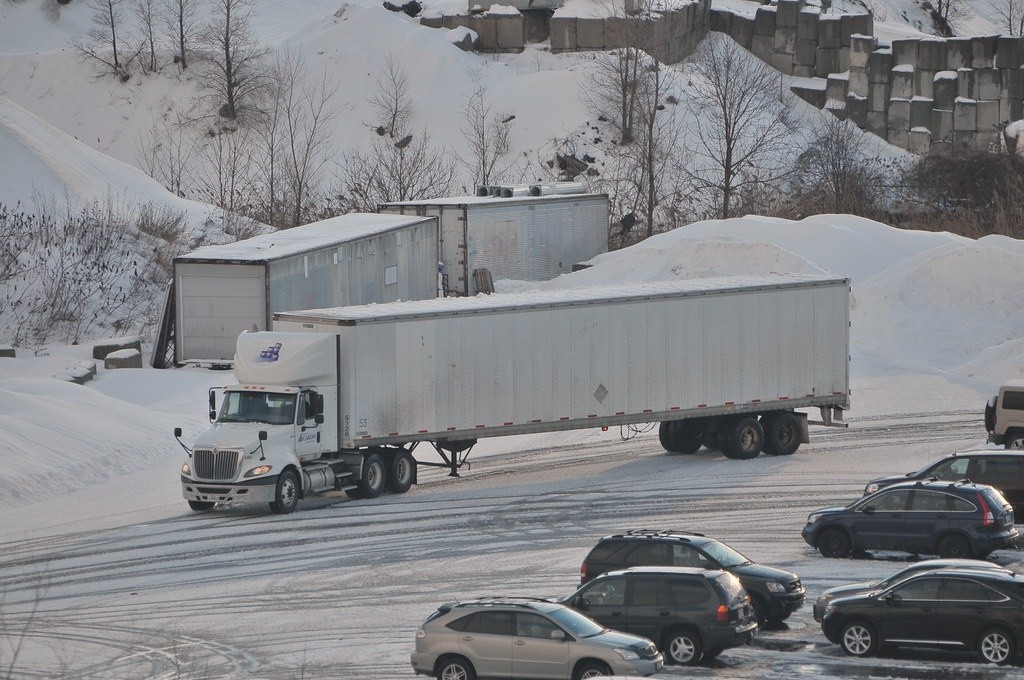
[820,570,1024,664]
[812,558,1024,624]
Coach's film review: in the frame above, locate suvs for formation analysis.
[410,596,665,680]
[799,475,1021,558]
[541,564,759,667]
[575,528,807,631]
[982,383,1024,451]
[862,450,1024,523]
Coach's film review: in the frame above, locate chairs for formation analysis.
[244,396,270,416]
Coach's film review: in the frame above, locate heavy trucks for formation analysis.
[171,273,855,518]
